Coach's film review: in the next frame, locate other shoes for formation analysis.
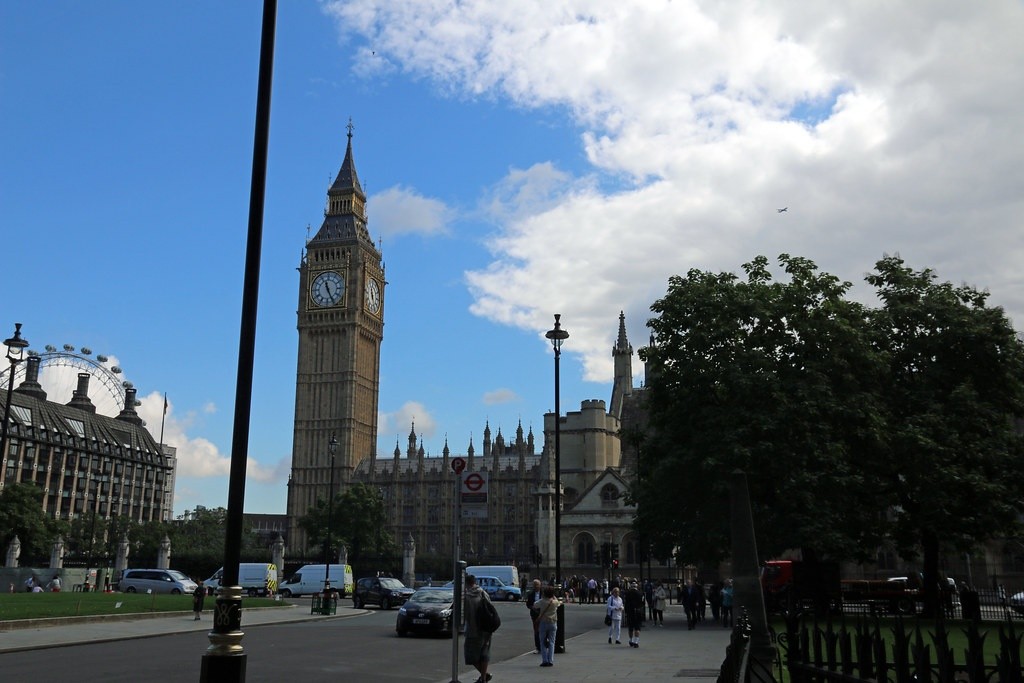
[195,617,200,621]
[475,673,492,683]
[629,641,639,648]
[616,640,621,644]
[652,623,657,627]
[534,650,540,654]
[539,661,553,667]
[608,637,611,644]
[658,623,664,627]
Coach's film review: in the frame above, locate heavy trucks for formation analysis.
[733,560,911,615]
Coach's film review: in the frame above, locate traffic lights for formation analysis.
[593,551,600,565]
[611,543,619,558]
[601,543,608,563]
[615,560,618,568]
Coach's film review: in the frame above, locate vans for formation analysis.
[202,563,277,597]
[278,565,353,598]
[117,568,198,595]
[868,577,961,612]
[443,566,519,588]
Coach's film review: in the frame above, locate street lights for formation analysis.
[324,432,340,589]
[546,314,569,654]
[104,506,118,592]
[0,324,29,474]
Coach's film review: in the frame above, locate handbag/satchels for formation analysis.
[192,596,197,603]
[650,600,654,608]
[604,615,612,625]
[477,591,501,632]
[534,617,540,632]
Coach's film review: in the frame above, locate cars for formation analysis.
[352,578,416,610]
[396,586,453,639]
[662,579,684,588]
[476,576,522,602]
[1009,592,1024,614]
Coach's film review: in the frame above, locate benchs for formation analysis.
[74,583,118,592]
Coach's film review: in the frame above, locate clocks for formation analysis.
[366,278,382,315]
[309,270,344,308]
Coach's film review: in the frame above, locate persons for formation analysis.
[464,575,501,683]
[999,583,1008,611]
[189,576,198,584]
[519,573,652,605]
[376,569,393,578]
[644,578,666,627]
[25,574,62,592]
[193,581,206,620]
[681,579,735,631]
[526,580,563,666]
[607,579,645,648]
[427,575,432,587]
[959,581,969,592]
